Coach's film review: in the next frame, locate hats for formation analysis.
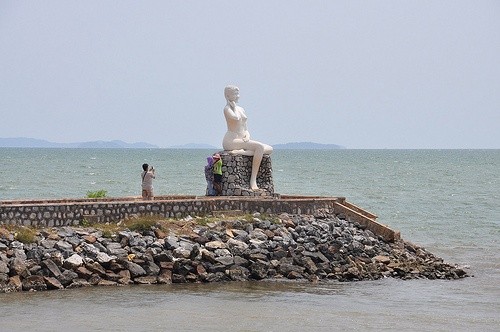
[213,154,221,158]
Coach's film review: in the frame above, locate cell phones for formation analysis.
[151,166,153,169]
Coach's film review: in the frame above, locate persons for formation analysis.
[204,156,213,195]
[211,153,224,196]
[141,162,156,200]
[222,86,274,192]
